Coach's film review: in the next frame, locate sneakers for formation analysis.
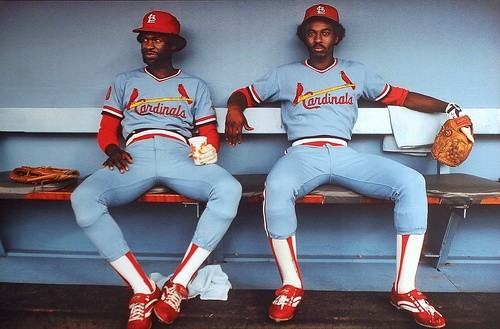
[390,282,446,328]
[154,279,188,325]
[269,285,304,321]
[127,281,161,329]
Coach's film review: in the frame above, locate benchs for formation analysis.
[0,108,500,269]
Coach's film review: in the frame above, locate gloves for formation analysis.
[445,102,474,143]
[189,145,218,165]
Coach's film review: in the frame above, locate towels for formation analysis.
[383,105,453,157]
[147,264,232,301]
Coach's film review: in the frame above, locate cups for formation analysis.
[188,137,208,166]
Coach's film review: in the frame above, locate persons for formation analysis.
[71,10,243,329]
[225,2,475,329]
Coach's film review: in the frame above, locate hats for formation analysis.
[304,4,339,23]
[132,11,187,51]
